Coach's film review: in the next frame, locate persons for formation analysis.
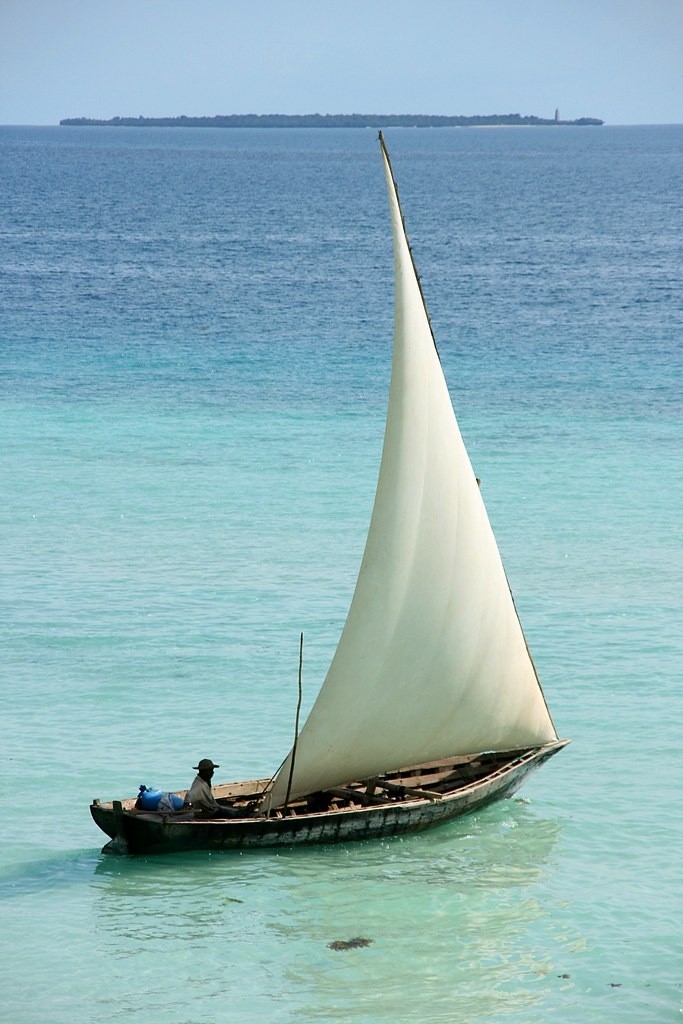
[181,758,258,822]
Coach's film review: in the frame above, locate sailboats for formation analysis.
[84,126,576,862]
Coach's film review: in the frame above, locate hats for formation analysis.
[193,759,219,770]
